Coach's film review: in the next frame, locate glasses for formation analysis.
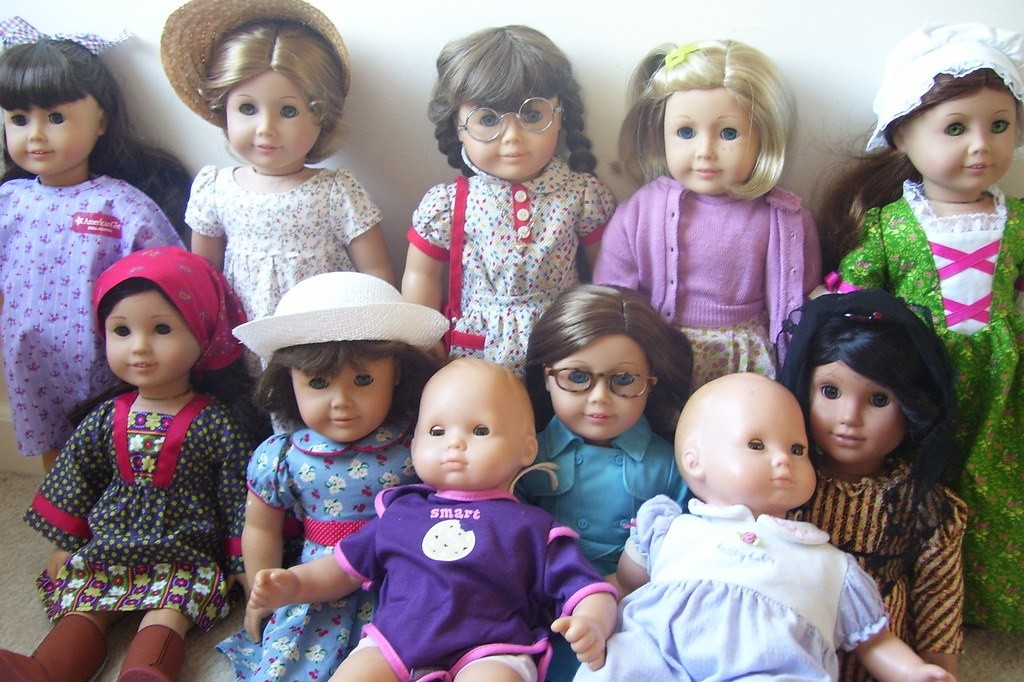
[454,97,563,143]
[545,367,656,398]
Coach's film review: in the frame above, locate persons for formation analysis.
[0,0,1024,682]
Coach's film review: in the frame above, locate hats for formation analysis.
[159,0,352,130]
[866,21,1024,151]
[232,271,453,361]
